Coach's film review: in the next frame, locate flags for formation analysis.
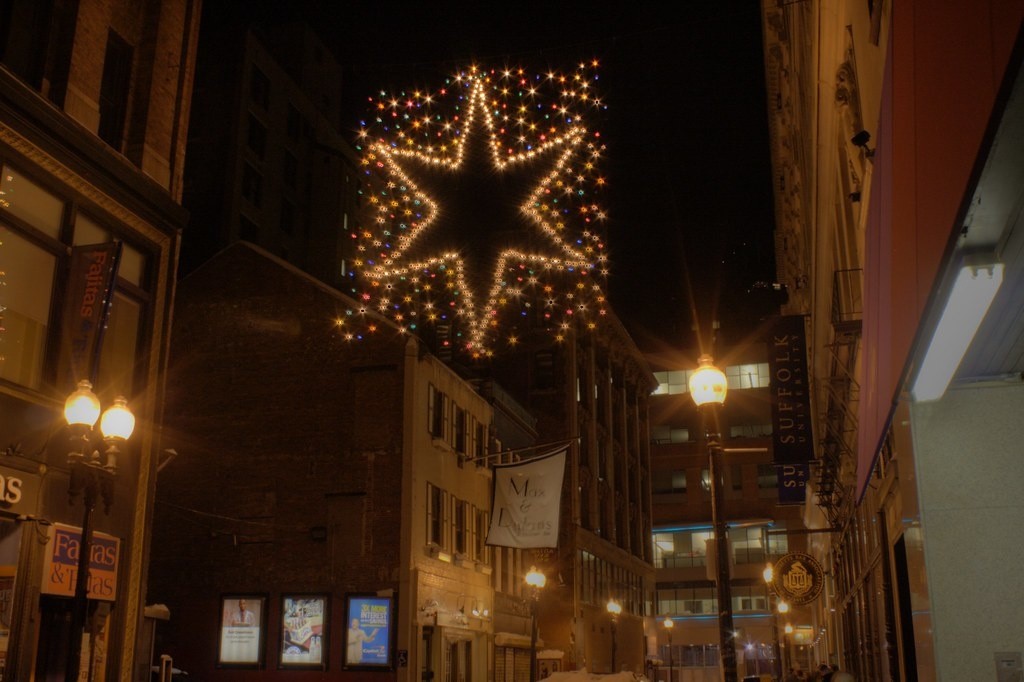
[484,443,570,549]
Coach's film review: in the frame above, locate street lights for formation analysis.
[525,564,546,682]
[763,562,783,682]
[663,616,674,682]
[59,376,133,682]
[606,597,622,674]
[687,353,738,682]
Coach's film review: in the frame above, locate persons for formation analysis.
[347,618,376,663]
[231,599,254,626]
[786,663,855,682]
